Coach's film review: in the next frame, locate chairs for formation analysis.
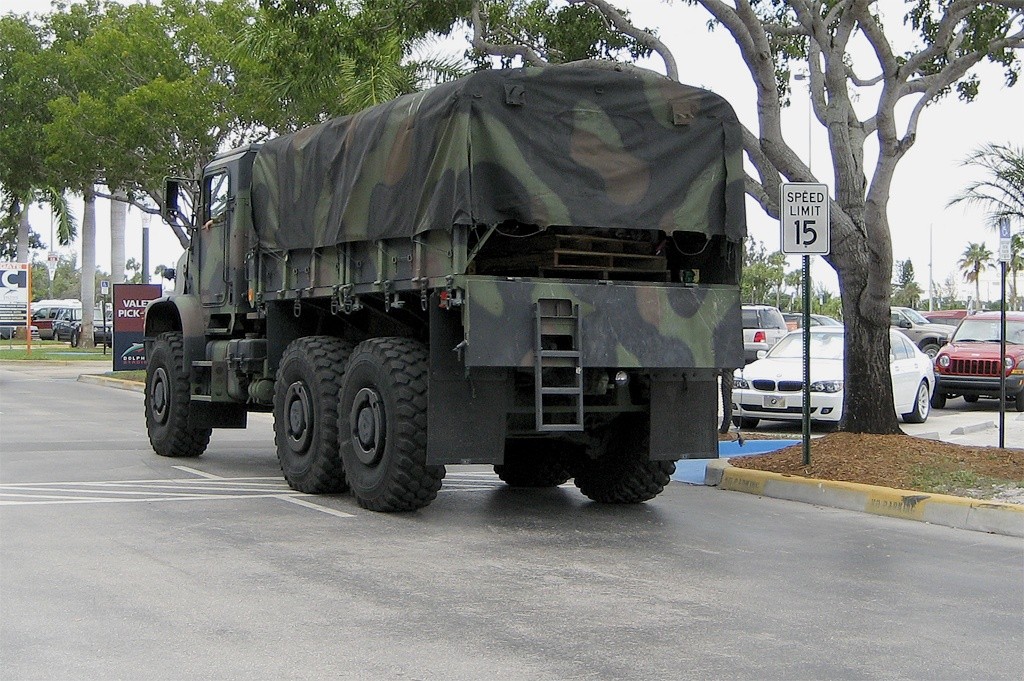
[969,324,992,339]
[52,312,56,317]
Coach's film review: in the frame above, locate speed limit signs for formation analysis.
[779,181,831,256]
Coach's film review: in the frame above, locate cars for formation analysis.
[729,302,981,370]
[730,323,936,430]
[30,298,113,348]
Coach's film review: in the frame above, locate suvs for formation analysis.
[928,309,1024,413]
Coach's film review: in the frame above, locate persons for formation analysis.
[205,213,224,230]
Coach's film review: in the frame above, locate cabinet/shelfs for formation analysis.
[476,234,673,281]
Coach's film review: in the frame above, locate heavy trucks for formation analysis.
[142,57,750,514]
